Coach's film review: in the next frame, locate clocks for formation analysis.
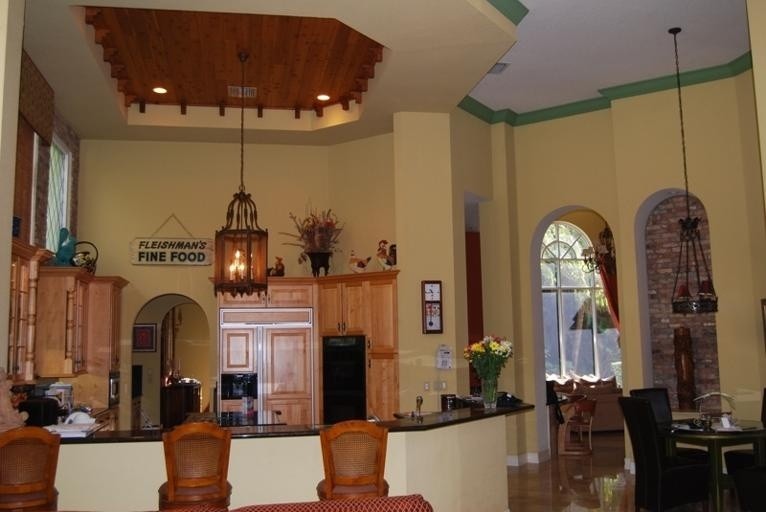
[421,279,444,335]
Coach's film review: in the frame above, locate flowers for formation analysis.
[463,335,512,400]
[282,204,349,256]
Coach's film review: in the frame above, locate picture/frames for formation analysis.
[131,323,158,354]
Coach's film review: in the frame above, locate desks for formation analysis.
[657,417,766,510]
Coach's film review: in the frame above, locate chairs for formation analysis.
[726,447,765,506]
[159,421,232,511]
[757,383,766,423]
[0,427,63,512]
[548,391,596,452]
[317,420,392,500]
[618,385,710,512]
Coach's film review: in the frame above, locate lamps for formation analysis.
[581,221,617,276]
[667,26,721,316]
[210,47,270,299]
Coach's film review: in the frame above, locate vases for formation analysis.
[482,380,499,414]
[302,246,332,279]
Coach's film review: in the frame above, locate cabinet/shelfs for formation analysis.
[365,268,401,423]
[317,269,367,335]
[211,274,321,429]
[165,379,201,424]
[32,264,88,378]
[10,235,50,384]
[85,274,127,424]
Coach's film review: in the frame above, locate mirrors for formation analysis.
[128,292,212,435]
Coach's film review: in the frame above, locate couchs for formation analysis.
[563,378,627,430]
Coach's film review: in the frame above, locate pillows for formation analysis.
[595,378,615,386]
[576,381,590,389]
